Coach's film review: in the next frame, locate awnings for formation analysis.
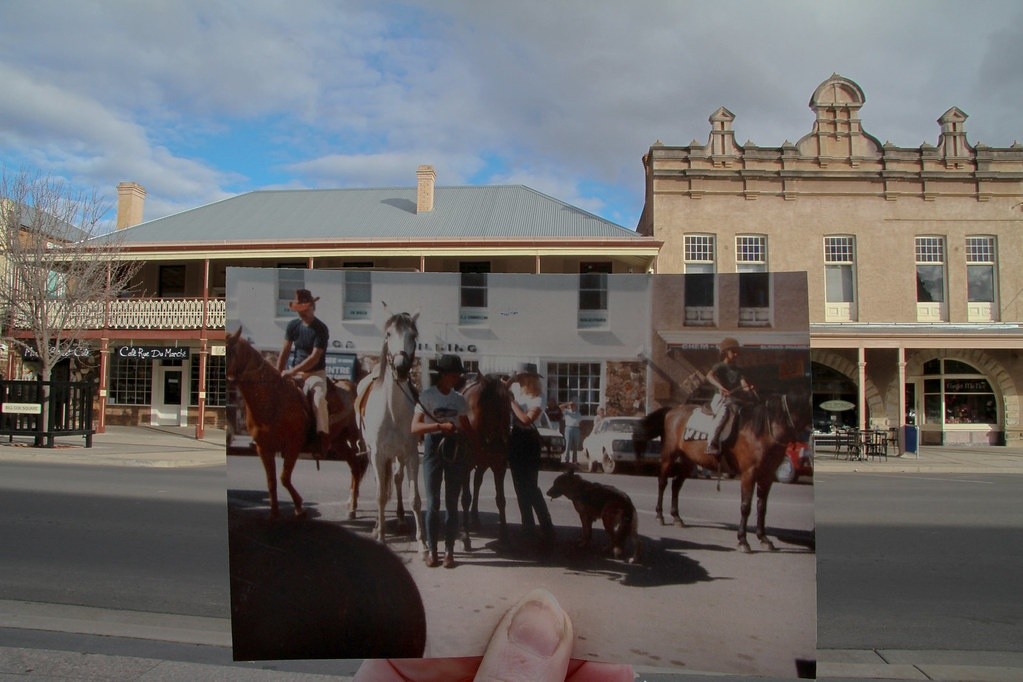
[658,328,809,350]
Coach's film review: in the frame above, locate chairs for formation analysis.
[833,430,898,461]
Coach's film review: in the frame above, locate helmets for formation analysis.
[719,337,740,354]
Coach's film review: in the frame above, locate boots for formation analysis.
[312,431,330,458]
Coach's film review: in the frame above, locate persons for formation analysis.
[276,288,331,457]
[705,338,743,455]
[504,363,554,546]
[594,408,604,425]
[411,354,468,566]
[559,401,580,467]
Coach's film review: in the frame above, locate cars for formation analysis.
[582,416,662,475]
[773,439,813,483]
[507,408,563,468]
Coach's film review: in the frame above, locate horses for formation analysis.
[226,301,812,568]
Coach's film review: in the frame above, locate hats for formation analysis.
[515,362,544,379]
[429,354,469,374]
[288,289,320,311]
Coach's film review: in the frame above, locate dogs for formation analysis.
[548,465,639,564]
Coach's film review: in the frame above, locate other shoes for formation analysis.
[443,550,455,569]
[515,529,535,537]
[704,443,720,455]
[536,528,553,537]
[425,549,439,568]
